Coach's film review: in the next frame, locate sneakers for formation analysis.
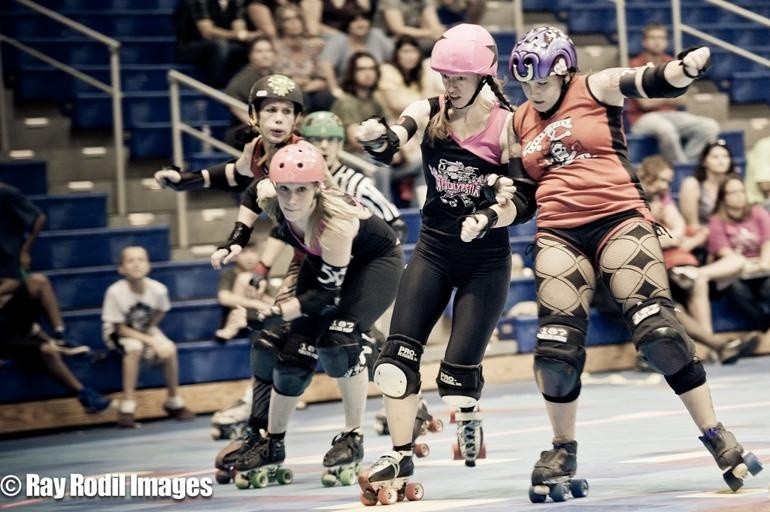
[49,332,91,355]
[718,330,762,365]
[163,404,195,420]
[118,408,135,428]
[83,397,119,416]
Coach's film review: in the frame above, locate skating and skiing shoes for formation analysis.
[700,424,763,492]
[529,437,588,503]
[210,393,488,505]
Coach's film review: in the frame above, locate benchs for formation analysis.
[0,1,769,386]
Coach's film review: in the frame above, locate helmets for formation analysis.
[248,23,577,184]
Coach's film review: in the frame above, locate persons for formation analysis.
[1,183,48,276]
[183,0,770,373]
[0,274,119,418]
[154,72,446,491]
[101,246,197,429]
[356,21,517,505]
[458,23,762,501]
